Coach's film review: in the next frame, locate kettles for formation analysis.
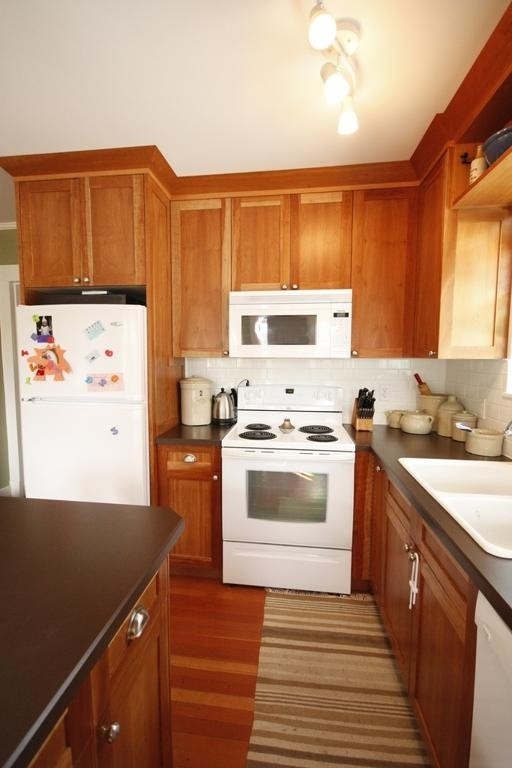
[212,388,239,424]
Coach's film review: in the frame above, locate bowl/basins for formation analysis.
[480,126,512,166]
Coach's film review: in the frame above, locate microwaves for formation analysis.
[228,290,353,360]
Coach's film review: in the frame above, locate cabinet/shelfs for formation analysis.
[380,481,476,768]
[352,187,417,358]
[64,551,173,768]
[170,198,231,356]
[418,145,511,361]
[157,444,222,579]
[232,191,354,290]
[18,172,170,287]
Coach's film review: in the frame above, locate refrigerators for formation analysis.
[12,302,152,508]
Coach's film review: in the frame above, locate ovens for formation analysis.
[221,448,356,596]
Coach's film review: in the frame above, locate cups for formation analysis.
[465,429,503,457]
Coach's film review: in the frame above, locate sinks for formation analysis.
[398,456,512,496]
[432,496,512,559]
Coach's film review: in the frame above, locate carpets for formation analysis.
[242,587,438,768]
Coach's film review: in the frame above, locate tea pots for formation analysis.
[384,408,403,429]
[398,410,435,435]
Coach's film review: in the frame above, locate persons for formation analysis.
[39,316,52,335]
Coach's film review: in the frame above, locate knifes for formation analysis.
[357,385,375,420]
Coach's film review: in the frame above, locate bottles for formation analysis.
[436,395,464,437]
[474,144,483,158]
[452,411,479,443]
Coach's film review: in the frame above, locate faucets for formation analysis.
[503,420,512,439]
